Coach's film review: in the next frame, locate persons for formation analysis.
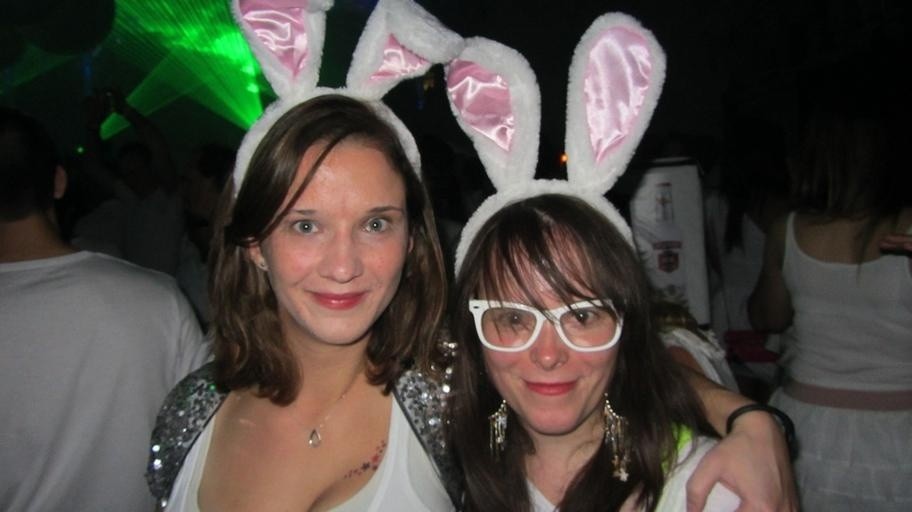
[442,176,745,510]
[143,85,802,512]
[702,188,794,387]
[736,95,911,511]
[0,109,214,512]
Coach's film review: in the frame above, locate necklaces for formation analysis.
[275,355,374,450]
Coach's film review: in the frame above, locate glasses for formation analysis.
[467,292,627,352]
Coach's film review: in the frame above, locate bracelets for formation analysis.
[727,402,795,446]
